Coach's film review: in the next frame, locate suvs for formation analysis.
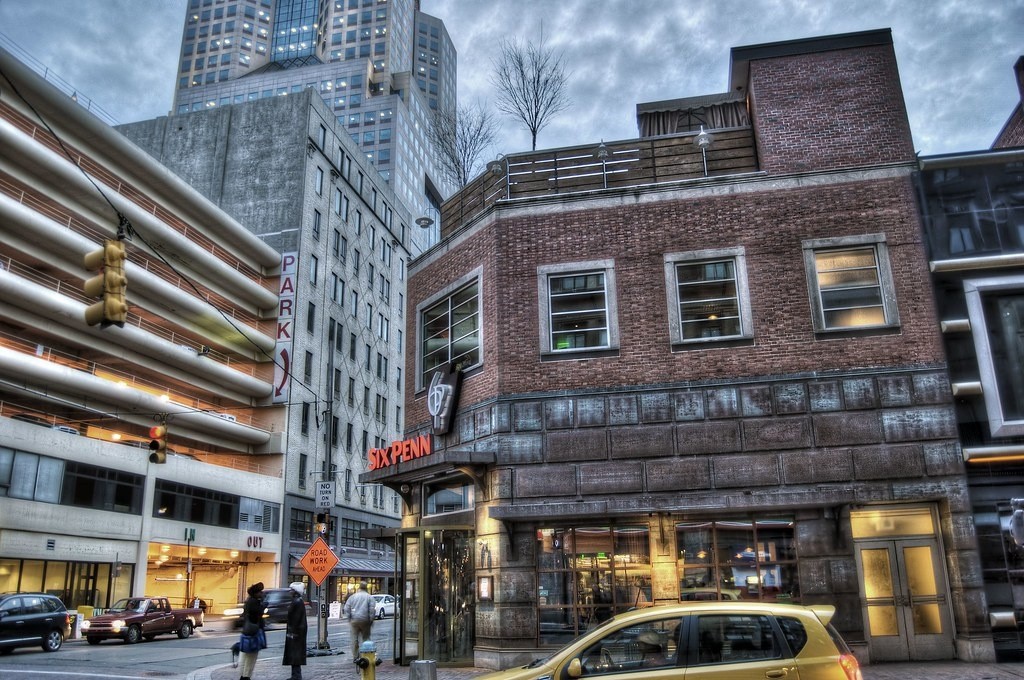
[0,593,71,655]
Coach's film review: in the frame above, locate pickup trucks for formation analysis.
[80,596,206,645]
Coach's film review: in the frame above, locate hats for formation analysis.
[247,581,264,596]
[289,582,305,596]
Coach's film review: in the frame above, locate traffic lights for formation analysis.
[148,425,166,467]
[85,239,126,332]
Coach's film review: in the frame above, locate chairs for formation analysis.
[698,631,720,664]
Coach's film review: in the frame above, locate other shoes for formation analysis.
[287,678,302,680]
[240,676,251,680]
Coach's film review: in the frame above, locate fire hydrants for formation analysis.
[356,640,382,680]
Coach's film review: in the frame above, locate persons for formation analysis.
[283,582,308,680]
[238,582,264,680]
[636,631,666,668]
[344,581,376,661]
[128,601,157,612]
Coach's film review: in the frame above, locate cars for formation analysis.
[472,600,863,680]
[681,589,743,602]
[372,594,401,620]
[228,588,310,631]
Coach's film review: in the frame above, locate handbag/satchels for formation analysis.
[230,642,241,656]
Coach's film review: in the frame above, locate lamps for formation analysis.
[485,153,510,198]
[400,483,413,494]
[477,538,489,548]
[694,125,713,177]
[590,137,612,188]
[416,206,440,228]
